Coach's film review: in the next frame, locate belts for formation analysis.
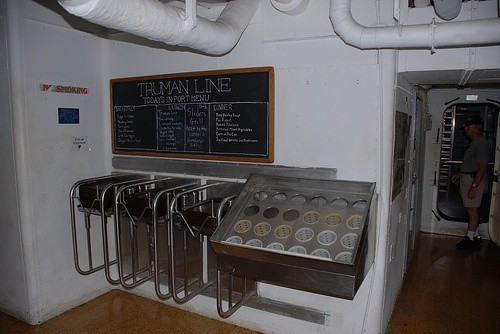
[460,172,477,174]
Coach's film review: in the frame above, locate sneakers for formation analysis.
[456,235,481,249]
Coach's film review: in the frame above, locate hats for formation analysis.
[461,116,483,127]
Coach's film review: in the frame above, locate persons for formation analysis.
[450,114,489,250]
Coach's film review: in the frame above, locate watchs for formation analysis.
[472,182,479,188]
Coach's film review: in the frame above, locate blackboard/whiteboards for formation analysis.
[110,66,275,164]
